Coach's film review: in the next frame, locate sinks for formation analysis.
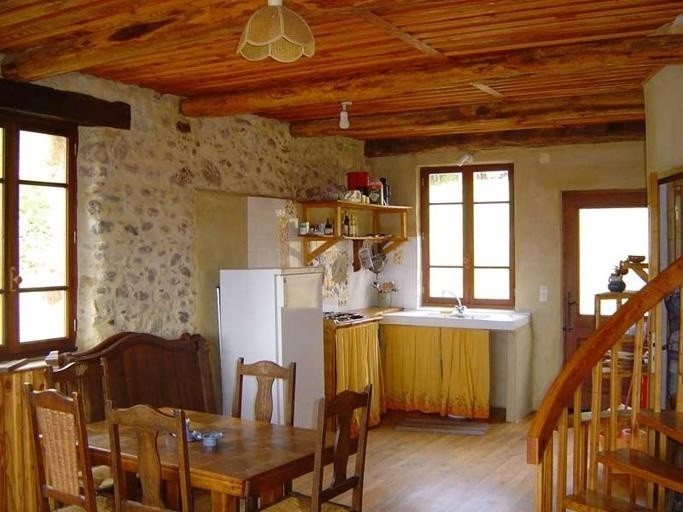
[448,313,490,321]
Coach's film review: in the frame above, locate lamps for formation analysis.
[335,100,353,132]
[233,0,317,64]
[453,151,474,168]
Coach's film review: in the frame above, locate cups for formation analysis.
[200,432,218,447]
[376,291,391,308]
[361,194,369,204]
[346,172,368,191]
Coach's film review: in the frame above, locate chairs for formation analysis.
[245,384,373,511]
[99,397,196,512]
[20,380,117,512]
[229,355,298,511]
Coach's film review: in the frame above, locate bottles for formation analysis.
[324,217,330,235]
[343,214,357,237]
[368,177,389,206]
[184,418,194,442]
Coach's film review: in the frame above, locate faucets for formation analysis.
[441,290,465,313]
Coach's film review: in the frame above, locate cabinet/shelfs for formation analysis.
[294,196,414,273]
[590,289,657,497]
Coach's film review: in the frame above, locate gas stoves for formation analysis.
[321,311,366,325]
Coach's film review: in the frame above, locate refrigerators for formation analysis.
[216,265,326,428]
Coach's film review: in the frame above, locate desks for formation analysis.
[35,406,360,512]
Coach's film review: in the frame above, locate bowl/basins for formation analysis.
[628,256,645,263]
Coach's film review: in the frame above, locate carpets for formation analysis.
[390,412,492,438]
